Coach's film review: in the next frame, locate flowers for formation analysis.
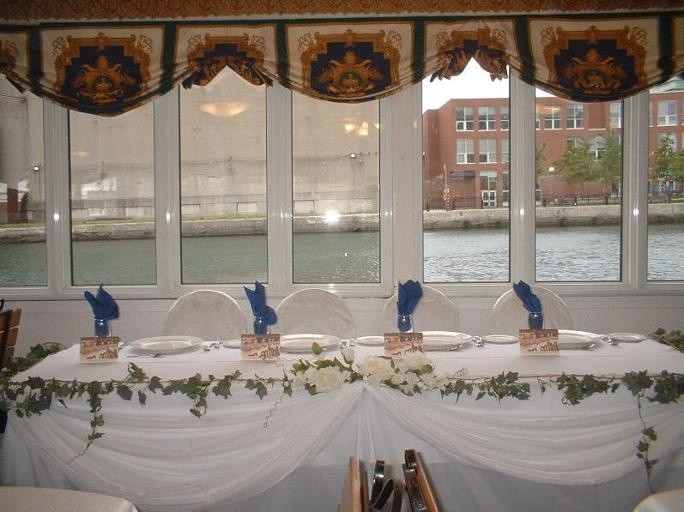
[0,325,684,485]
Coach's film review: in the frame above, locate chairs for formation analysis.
[382,284,461,337]
[161,289,246,342]
[338,447,439,512]
[0,298,22,370]
[268,288,355,340]
[488,285,573,335]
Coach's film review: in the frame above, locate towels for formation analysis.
[395,278,423,331]
[513,280,544,329]
[243,280,278,334]
[84,284,119,336]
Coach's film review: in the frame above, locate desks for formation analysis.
[0,334,684,512]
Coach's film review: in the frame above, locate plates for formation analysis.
[222,338,242,349]
[356,336,385,346]
[609,331,648,342]
[418,330,475,351]
[130,334,203,353]
[557,328,603,349]
[482,334,520,344]
[279,333,341,353]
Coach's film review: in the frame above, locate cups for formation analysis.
[253,315,270,334]
[398,315,413,334]
[92,319,113,337]
[528,312,545,330]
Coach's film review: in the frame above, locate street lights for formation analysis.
[33,167,41,202]
[549,167,554,202]
[351,153,356,199]
[422,152,428,211]
